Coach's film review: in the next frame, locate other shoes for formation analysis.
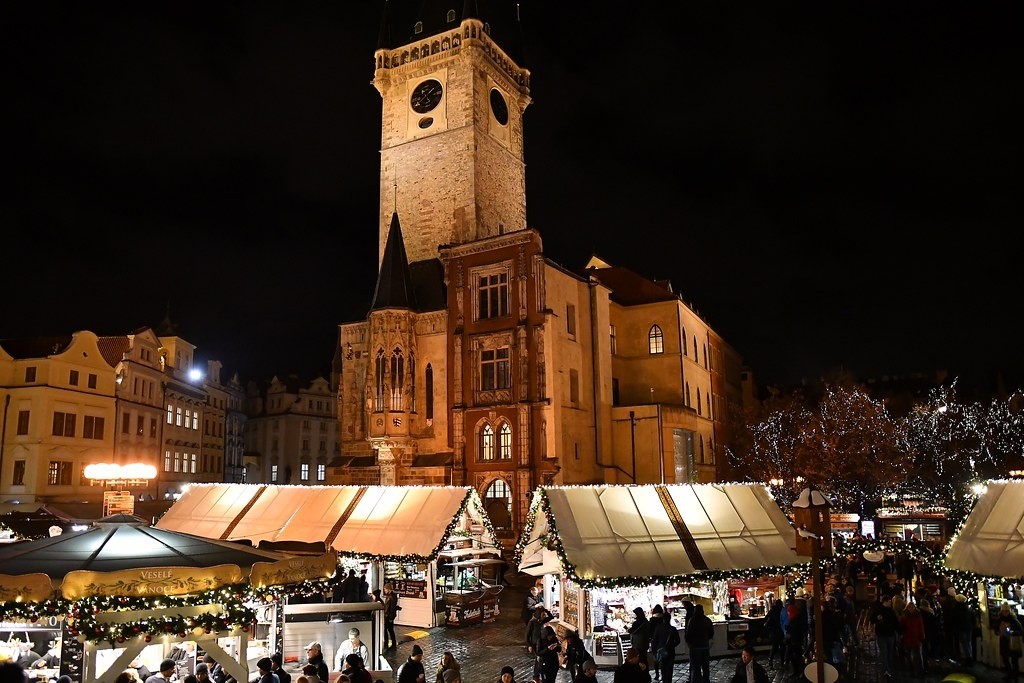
[653,674,659,680]
[685,641,1024,683]
[383,640,396,650]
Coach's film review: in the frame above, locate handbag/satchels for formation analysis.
[651,647,669,660]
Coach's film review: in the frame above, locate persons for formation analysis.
[0,532,1024,683]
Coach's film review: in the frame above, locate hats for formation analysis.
[693,604,702,608]
[202,652,215,662]
[303,641,321,651]
[270,651,281,666]
[257,657,269,671]
[564,630,574,637]
[681,596,691,601]
[442,668,459,683]
[195,662,207,674]
[626,647,638,661]
[582,660,599,669]
[412,643,422,655]
[160,659,174,672]
[383,582,391,590]
[346,654,359,664]
[501,666,514,676]
[529,586,538,591]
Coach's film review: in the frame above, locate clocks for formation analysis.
[410,79,443,114]
